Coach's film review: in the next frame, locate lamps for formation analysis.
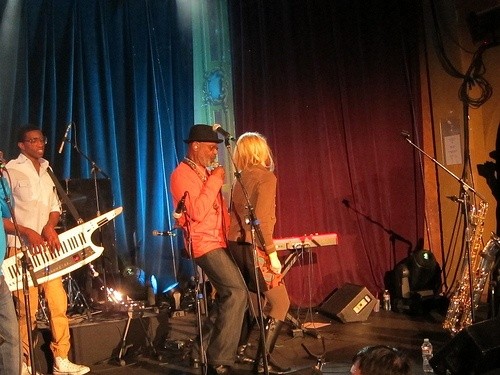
[163,282,184,318]
[394,249,442,301]
[121,265,156,307]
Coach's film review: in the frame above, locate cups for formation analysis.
[373,299,380,312]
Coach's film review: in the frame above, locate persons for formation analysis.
[169,124,249,375]
[2,125,89,375]
[1,151,29,375]
[222,131,291,374]
[349,345,409,375]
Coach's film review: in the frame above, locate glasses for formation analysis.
[208,145,219,152]
[25,137,48,146]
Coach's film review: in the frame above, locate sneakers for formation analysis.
[22,361,42,375]
[53,356,90,375]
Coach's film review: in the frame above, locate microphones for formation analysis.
[212,123,237,142]
[59,124,71,154]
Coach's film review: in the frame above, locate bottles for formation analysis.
[422,339,434,372]
[383,289,391,311]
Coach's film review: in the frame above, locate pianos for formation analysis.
[262,233,339,339]
[1,205,124,292]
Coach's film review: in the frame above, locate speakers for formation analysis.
[315,282,378,323]
[55,177,118,276]
[429,317,500,375]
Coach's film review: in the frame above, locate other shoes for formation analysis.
[209,362,229,375]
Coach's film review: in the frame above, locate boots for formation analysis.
[235,310,256,364]
[253,316,292,375]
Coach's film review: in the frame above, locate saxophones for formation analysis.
[442,195,500,337]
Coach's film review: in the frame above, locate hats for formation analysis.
[182,123,223,144]
